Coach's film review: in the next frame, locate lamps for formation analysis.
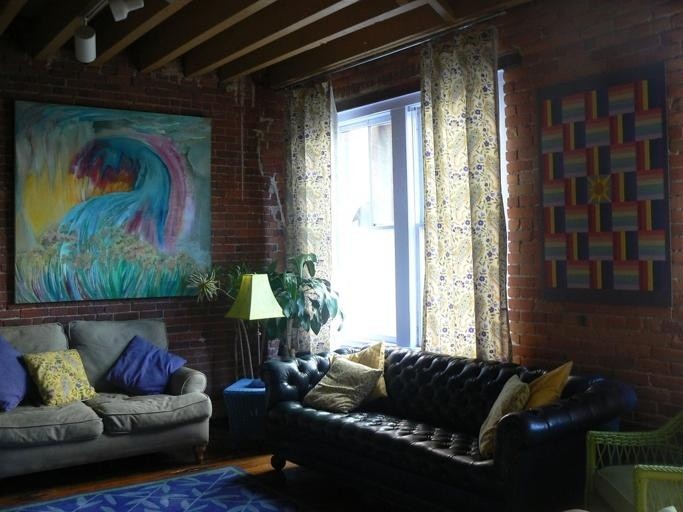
[225,273,285,387]
[74,0,145,63]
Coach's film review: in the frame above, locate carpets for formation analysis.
[0,466,309,512]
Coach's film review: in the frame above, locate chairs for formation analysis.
[586,408,683,512]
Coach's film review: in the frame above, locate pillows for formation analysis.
[525,359,574,409]
[478,375,530,456]
[0,336,33,411]
[106,335,188,396]
[304,358,383,413]
[22,349,97,404]
[334,342,389,399]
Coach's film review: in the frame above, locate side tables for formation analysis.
[223,379,267,459]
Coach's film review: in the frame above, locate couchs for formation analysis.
[265,347,638,512]
[0,319,214,478]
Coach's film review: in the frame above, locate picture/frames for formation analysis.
[536,60,675,307]
